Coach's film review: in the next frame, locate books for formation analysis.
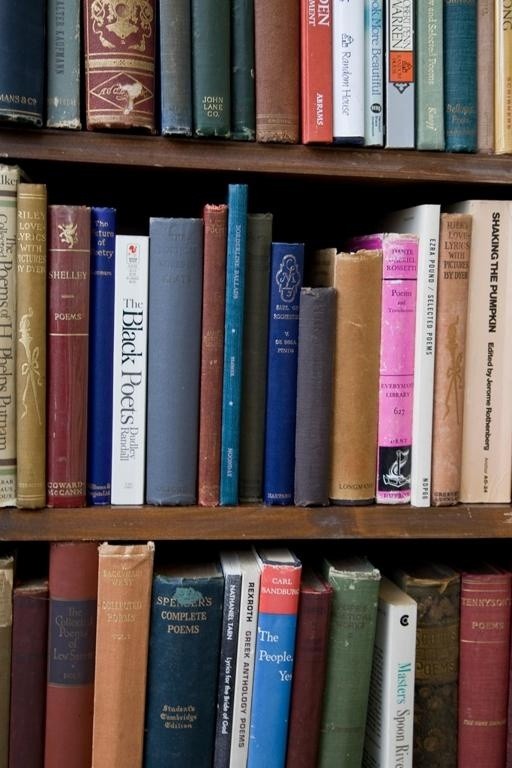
[0,541,512,768]
[1,163,512,509]
[2,0,511,155]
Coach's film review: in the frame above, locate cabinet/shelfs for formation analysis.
[0,122,510,542]
